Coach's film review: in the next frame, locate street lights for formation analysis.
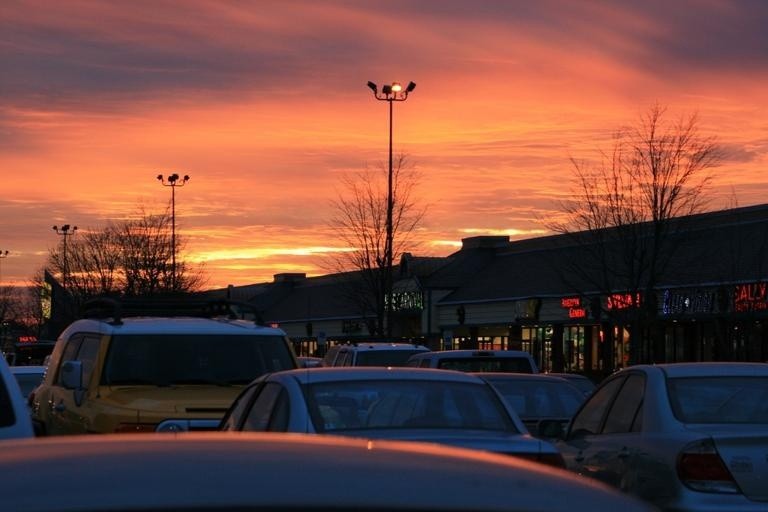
[156,173,190,312]
[367,79,416,335]
[53,225,78,316]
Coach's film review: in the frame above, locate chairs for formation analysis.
[310,394,462,430]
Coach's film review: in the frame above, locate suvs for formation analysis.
[27,295,303,440]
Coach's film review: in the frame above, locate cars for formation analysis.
[0,430,666,512]
[536,361,768,512]
[0,349,37,440]
[9,364,47,397]
[214,366,568,470]
[296,334,598,442]
